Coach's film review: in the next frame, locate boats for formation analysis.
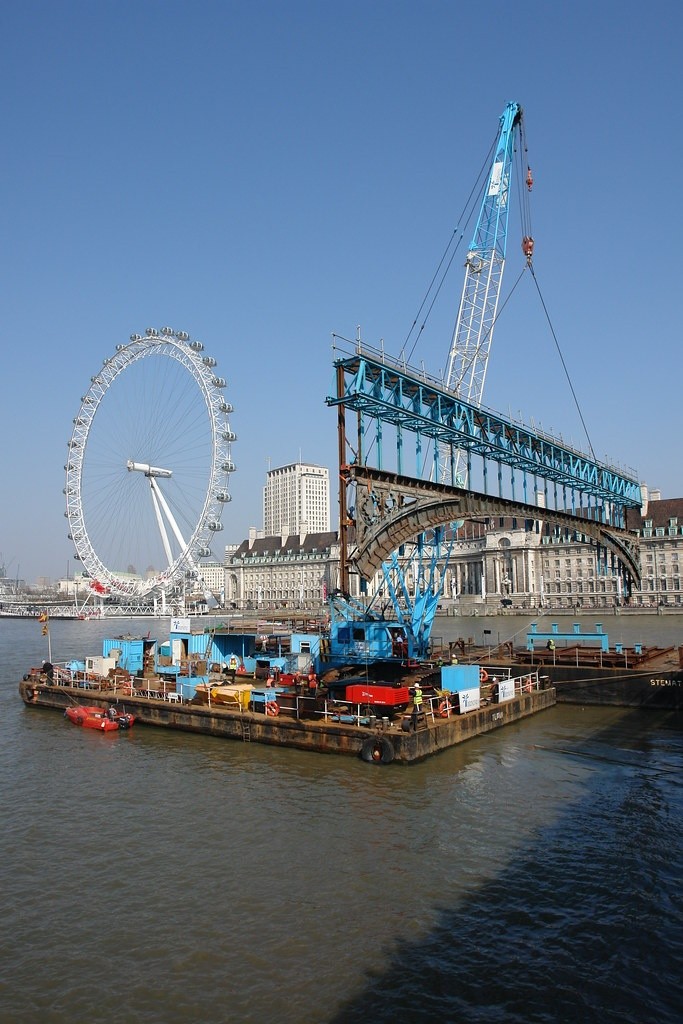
[64,705,135,733]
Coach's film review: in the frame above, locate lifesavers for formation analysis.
[478,669,489,683]
[266,701,280,718]
[438,699,453,719]
[121,680,134,697]
[526,680,533,693]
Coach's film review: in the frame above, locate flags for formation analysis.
[38,610,47,622]
[41,624,48,636]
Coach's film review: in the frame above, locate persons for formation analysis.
[408,683,423,723]
[396,634,405,657]
[436,654,460,667]
[265,669,318,697]
[144,647,151,664]
[108,705,117,721]
[260,635,269,651]
[228,656,237,684]
[546,639,556,651]
[41,659,54,686]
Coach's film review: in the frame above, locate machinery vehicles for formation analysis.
[251,98,611,671]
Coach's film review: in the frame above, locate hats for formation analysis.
[42,660,45,663]
[231,656,235,658]
[452,654,456,657]
[438,657,442,660]
[414,683,420,687]
